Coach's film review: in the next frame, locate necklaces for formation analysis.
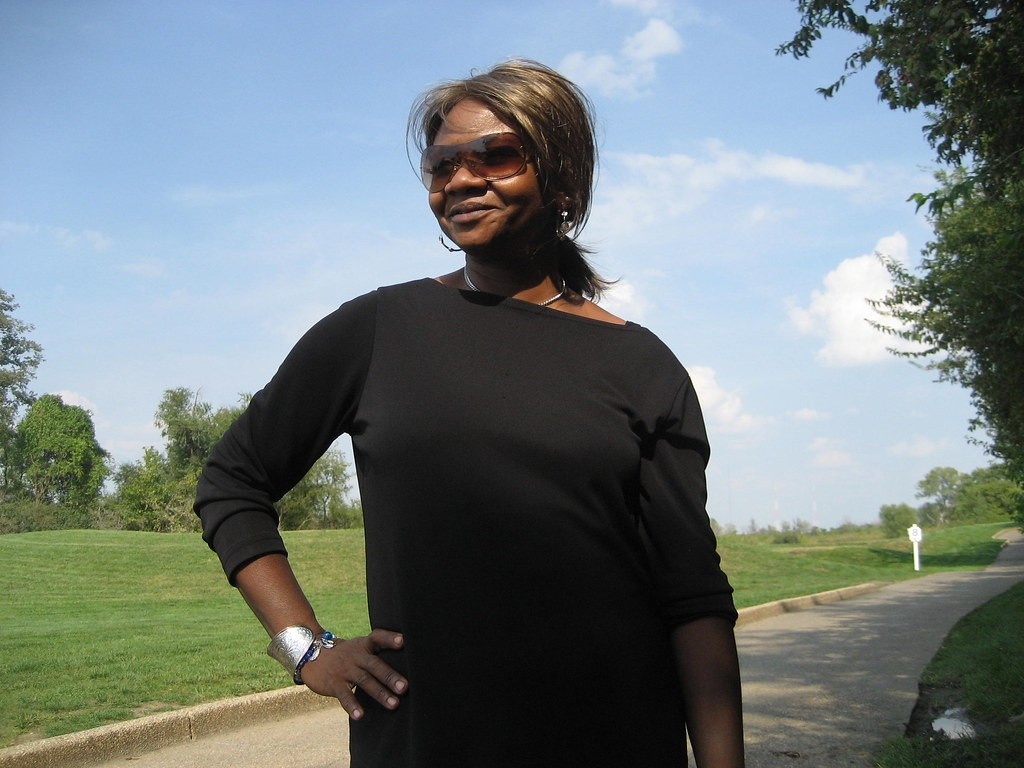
[463,262,567,307]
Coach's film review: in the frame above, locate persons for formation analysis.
[193,58,744,768]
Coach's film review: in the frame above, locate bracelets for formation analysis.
[265,625,336,687]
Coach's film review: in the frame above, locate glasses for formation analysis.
[421,132,534,194]
